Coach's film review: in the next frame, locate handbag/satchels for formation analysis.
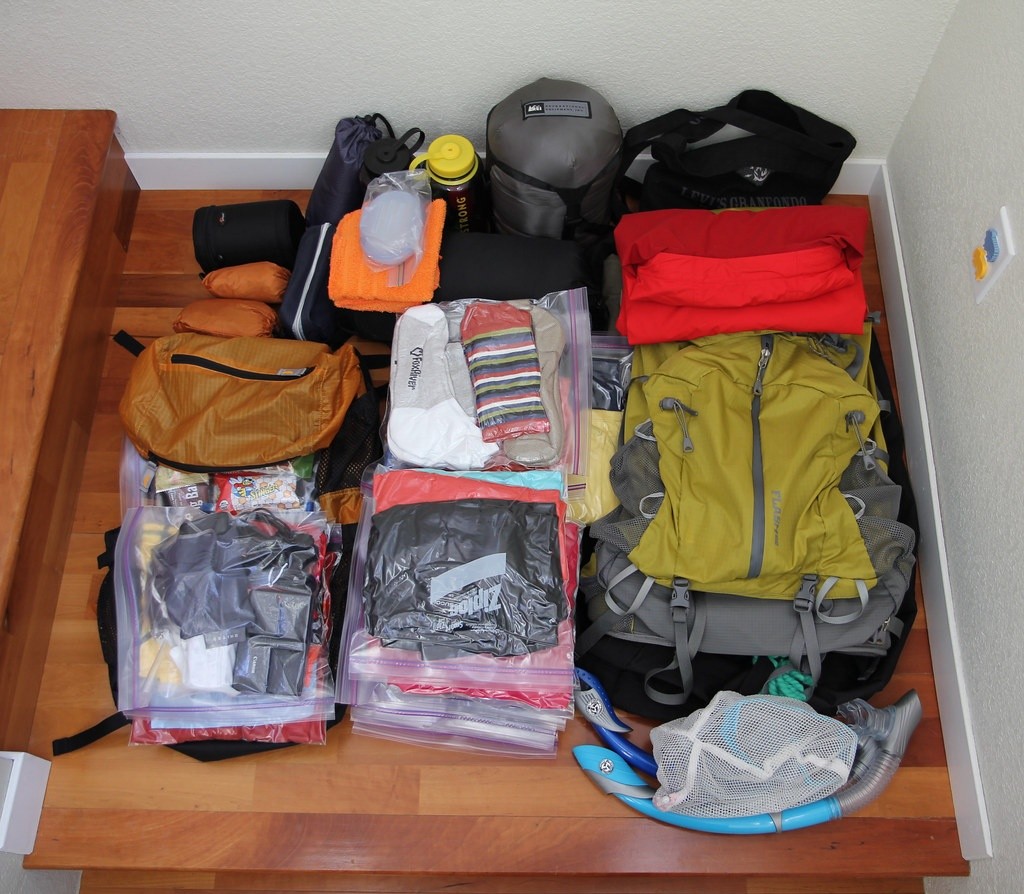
[51,77,919,832]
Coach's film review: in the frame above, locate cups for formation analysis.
[409,134,485,232]
